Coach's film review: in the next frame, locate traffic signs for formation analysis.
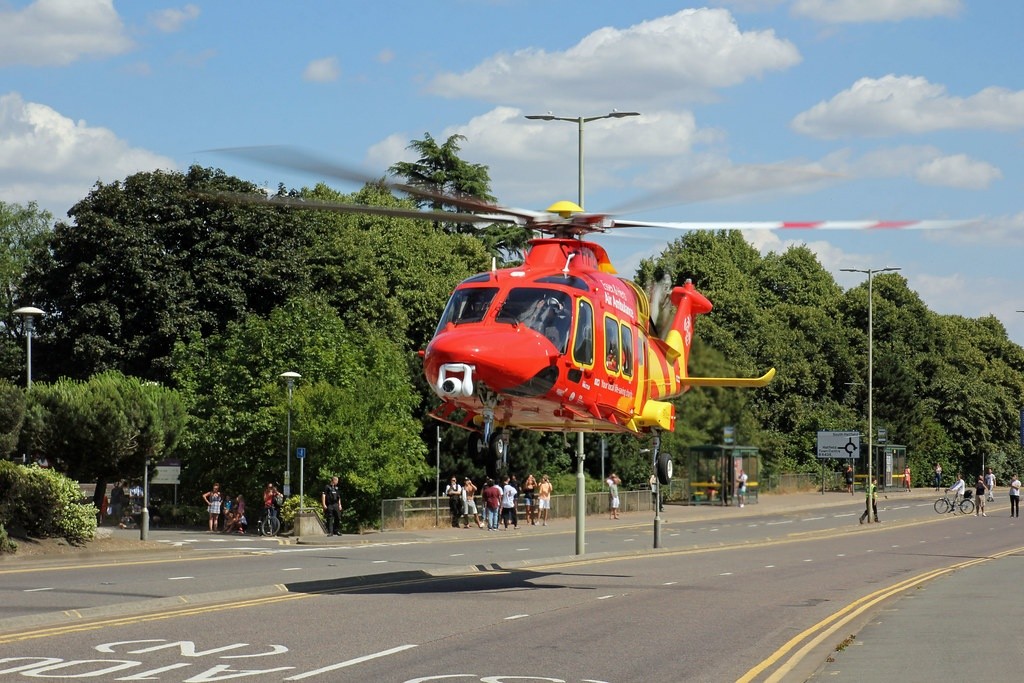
[817,431,860,458]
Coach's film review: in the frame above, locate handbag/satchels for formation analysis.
[273,491,284,506]
[107,504,112,515]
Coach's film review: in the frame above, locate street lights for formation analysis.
[839,266,902,523]
[12,306,47,391]
[525,111,643,554]
[279,371,302,533]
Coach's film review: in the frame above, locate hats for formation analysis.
[464,477,469,480]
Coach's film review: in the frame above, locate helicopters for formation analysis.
[182,144,985,486]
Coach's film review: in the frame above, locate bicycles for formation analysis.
[934,488,975,515]
[260,503,282,537]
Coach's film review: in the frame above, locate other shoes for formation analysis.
[875,517,879,522]
[614,517,619,520]
[337,531,342,536]
[859,517,863,524]
[452,520,456,527]
[609,517,612,520]
[479,524,483,528]
[982,513,986,517]
[464,524,469,528]
[327,533,333,537]
[456,522,460,528]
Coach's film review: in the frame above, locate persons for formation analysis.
[934,462,942,492]
[1007,474,1021,518]
[902,464,911,492]
[974,475,987,517]
[223,494,248,534]
[203,482,223,532]
[708,475,721,502]
[603,473,622,521]
[844,465,853,493]
[321,476,343,537]
[650,475,664,513]
[446,473,552,531]
[984,468,996,502]
[262,483,283,537]
[735,470,749,508]
[98,481,163,534]
[859,478,881,525]
[946,473,965,515]
